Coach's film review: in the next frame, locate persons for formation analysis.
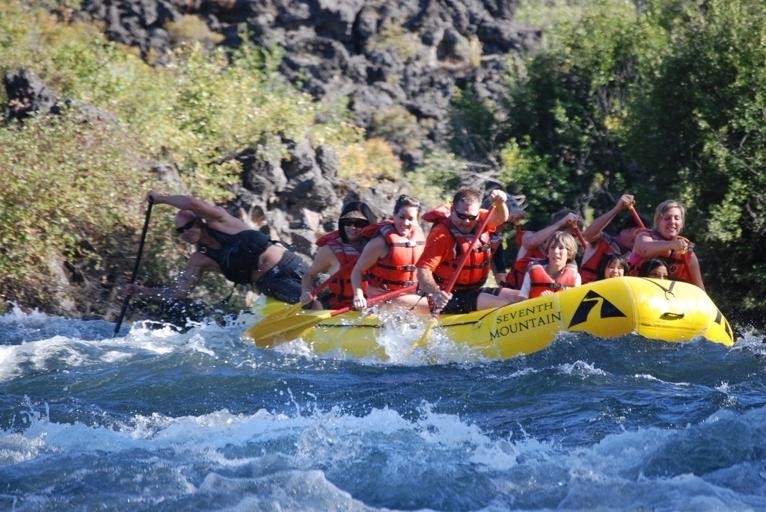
[123,191,331,308]
[415,189,521,314]
[300,202,380,310]
[506,194,705,299]
[351,196,427,310]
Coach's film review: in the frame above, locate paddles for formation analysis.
[403,197,499,358]
[249,251,419,347]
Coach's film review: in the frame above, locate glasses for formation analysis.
[455,209,477,221]
[339,217,367,228]
[176,218,195,234]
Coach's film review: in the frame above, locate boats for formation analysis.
[253,277,735,361]
